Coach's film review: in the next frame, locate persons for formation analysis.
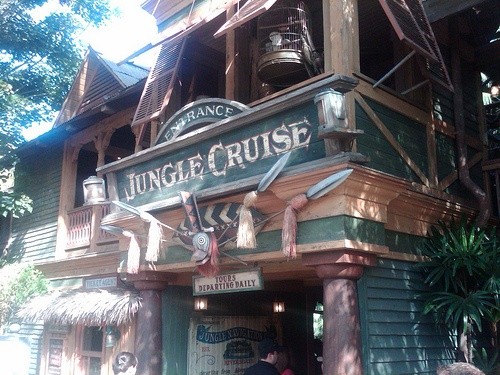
[436,362,485,375]
[112,352,138,375]
[244,338,281,375]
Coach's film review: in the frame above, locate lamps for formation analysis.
[194,295,208,314]
[105,326,120,349]
[82,175,110,207]
[491,82,500,100]
[314,87,348,129]
[272,295,287,319]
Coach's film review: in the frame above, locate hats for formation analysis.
[259,339,285,353]
